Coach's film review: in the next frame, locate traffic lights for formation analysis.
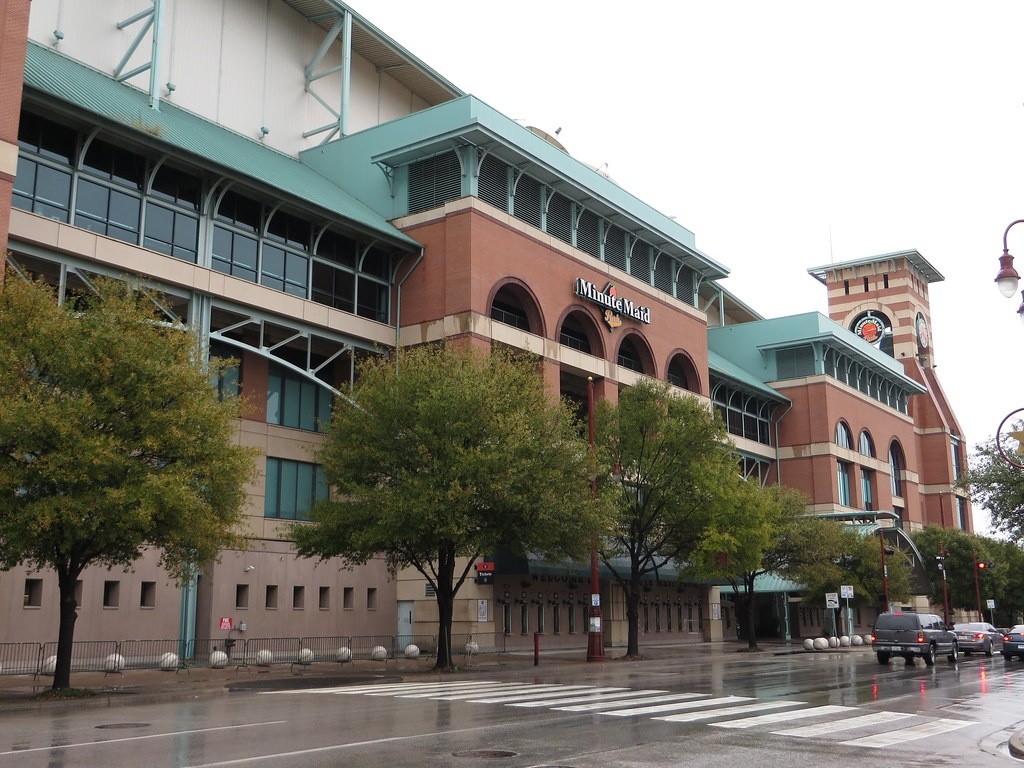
[976,562,995,569]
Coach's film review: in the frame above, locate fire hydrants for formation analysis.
[534,631,541,666]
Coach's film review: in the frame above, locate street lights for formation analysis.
[586,446,622,662]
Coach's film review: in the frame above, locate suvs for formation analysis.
[871,611,958,666]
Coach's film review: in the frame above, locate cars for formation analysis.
[953,622,1005,657]
[1004,625,1024,661]
